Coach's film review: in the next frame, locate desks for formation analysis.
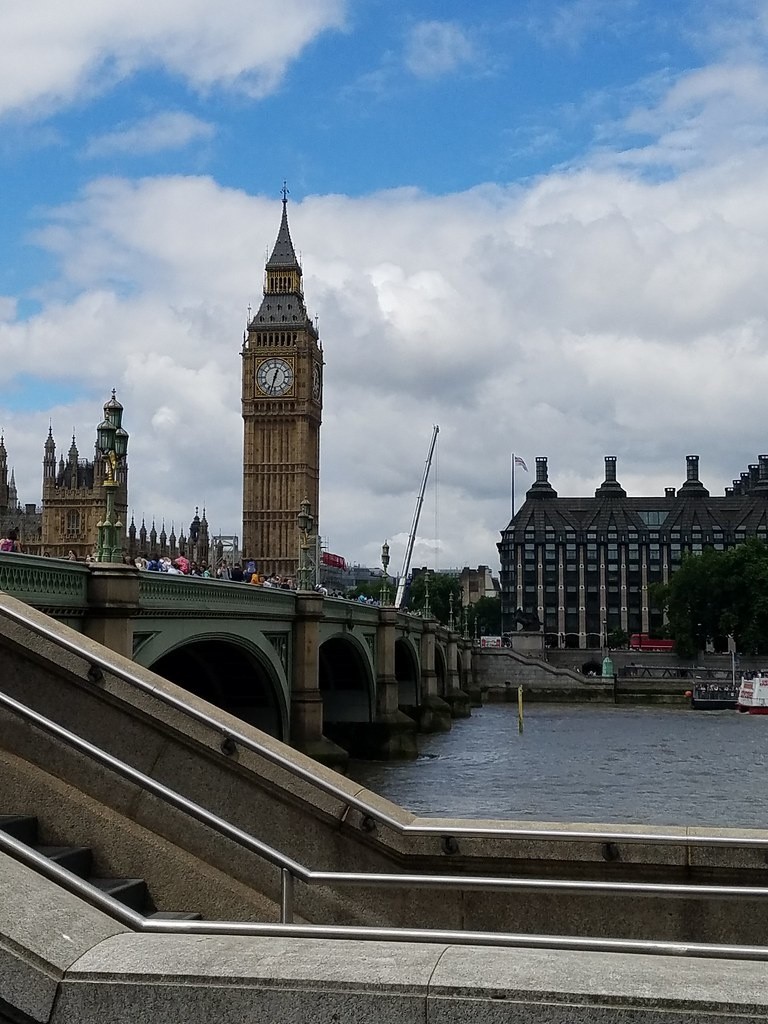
[624,666,762,678]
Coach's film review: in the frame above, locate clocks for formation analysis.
[255,357,294,397]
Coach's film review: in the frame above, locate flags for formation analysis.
[514,457,528,472]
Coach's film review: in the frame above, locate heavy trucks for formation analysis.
[630,633,677,654]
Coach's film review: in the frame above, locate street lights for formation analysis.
[379,539,392,607]
[422,570,433,618]
[463,607,470,639]
[449,590,455,630]
[296,495,318,591]
[91,388,129,563]
[637,586,643,654]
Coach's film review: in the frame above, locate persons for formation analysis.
[314,581,380,605]
[0,537,23,553]
[43,547,50,557]
[697,683,739,700]
[525,652,600,677]
[756,670,762,678]
[400,605,423,617]
[68,549,96,562]
[122,550,297,589]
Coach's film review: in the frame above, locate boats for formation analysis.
[735,672,768,715]
